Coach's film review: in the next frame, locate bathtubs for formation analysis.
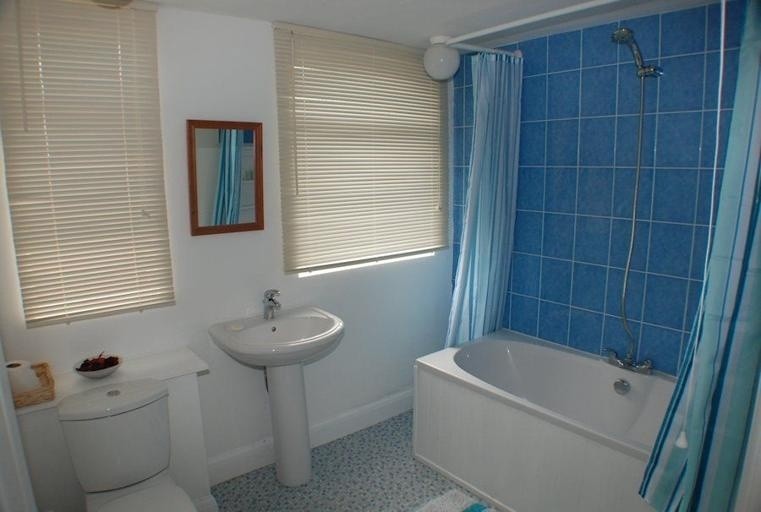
[412,329,708,512]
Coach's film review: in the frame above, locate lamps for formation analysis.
[424,33,460,83]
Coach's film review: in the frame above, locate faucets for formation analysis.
[262,298,284,322]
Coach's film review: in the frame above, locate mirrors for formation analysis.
[186,119,264,236]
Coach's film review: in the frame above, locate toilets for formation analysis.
[54,378,195,512]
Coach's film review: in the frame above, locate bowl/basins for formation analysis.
[73,353,123,379]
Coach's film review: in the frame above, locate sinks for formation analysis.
[207,308,344,367]
